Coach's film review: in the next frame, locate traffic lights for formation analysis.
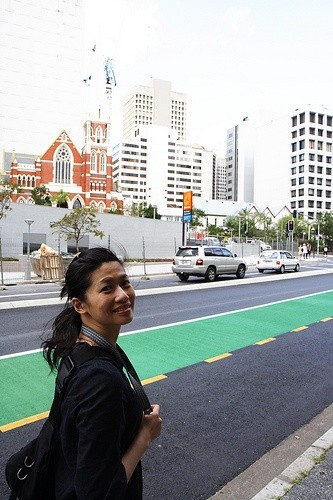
[288,220,294,232]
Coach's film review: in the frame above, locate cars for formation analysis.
[256,250,301,273]
[246,241,271,251]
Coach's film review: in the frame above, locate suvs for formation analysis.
[171,245,248,282]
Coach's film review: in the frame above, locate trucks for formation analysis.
[186,236,220,246]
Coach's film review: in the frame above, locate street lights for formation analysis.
[24,219,34,280]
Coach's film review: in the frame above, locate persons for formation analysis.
[324,246,327,255]
[41,247,161,500]
[298,242,311,259]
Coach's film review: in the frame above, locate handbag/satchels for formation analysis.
[6,420,56,500]
